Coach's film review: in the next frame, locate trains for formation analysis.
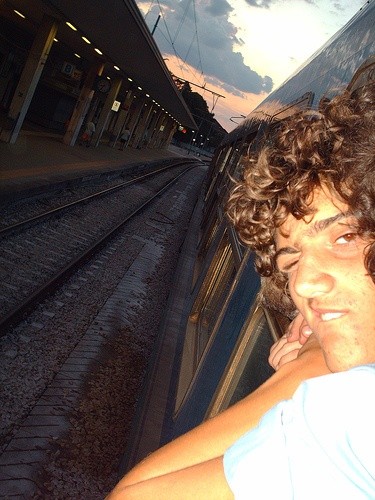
[113,0,375,482]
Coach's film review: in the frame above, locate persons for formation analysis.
[106,61,375,500]
[79,118,96,147]
[117,127,130,151]
